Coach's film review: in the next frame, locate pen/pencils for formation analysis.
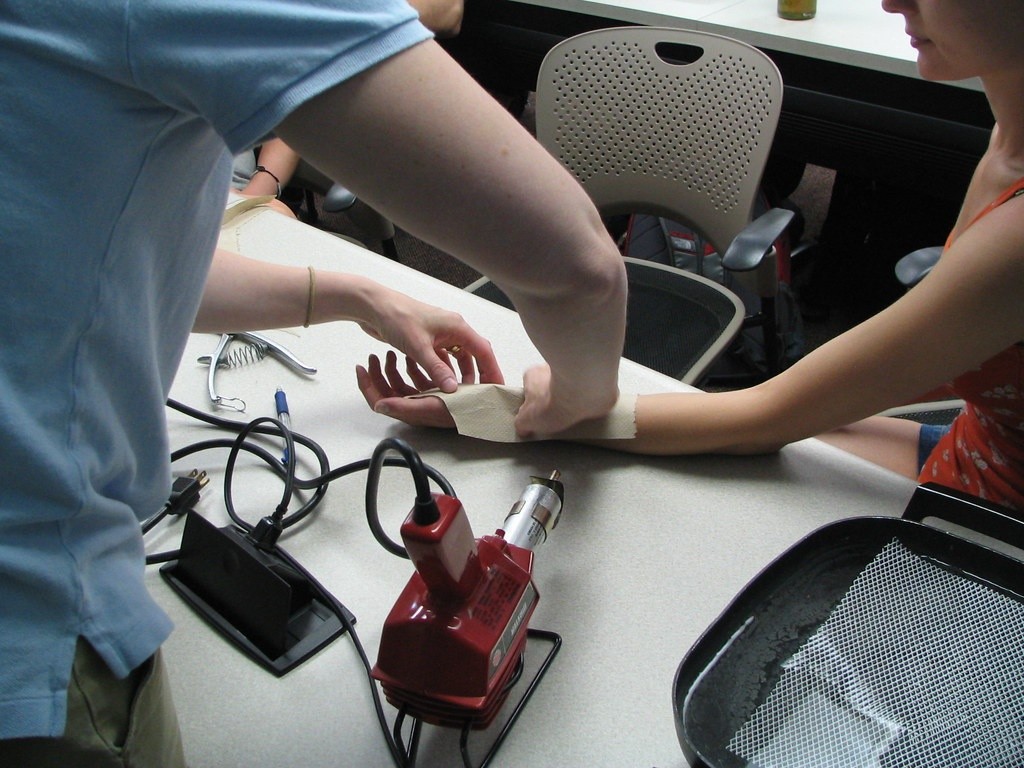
[274,385,297,467]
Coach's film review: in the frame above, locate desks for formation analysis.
[437,0,996,320]
[140,206,920,768]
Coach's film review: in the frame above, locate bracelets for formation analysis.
[251,165,282,197]
[304,265,314,328]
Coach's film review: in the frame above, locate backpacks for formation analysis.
[623,183,804,379]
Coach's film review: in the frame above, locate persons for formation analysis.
[354,0,1024,515]
[227,138,302,220]
[0,0,627,768]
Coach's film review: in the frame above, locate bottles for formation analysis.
[778,0,816,20]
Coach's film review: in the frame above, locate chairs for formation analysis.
[463,26,799,383]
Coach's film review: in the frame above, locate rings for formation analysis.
[447,345,463,355]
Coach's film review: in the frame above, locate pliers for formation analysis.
[196,333,319,413]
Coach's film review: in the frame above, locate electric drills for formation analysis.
[364,469,565,768]
